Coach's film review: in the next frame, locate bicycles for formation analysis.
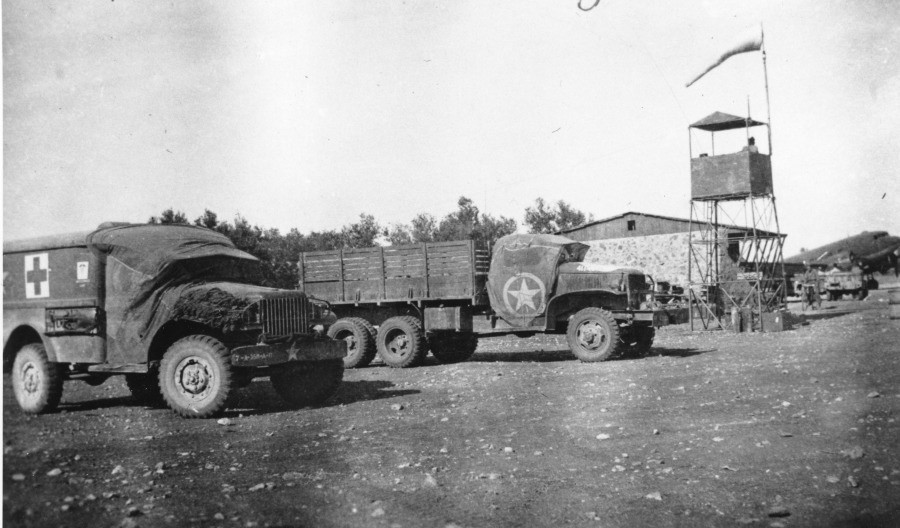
[801,284,823,313]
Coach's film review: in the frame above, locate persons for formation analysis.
[802,263,821,308]
[747,137,758,154]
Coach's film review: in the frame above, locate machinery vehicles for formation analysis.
[825,270,874,301]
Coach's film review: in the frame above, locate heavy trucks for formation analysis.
[296,232,659,369]
[4,221,349,420]
